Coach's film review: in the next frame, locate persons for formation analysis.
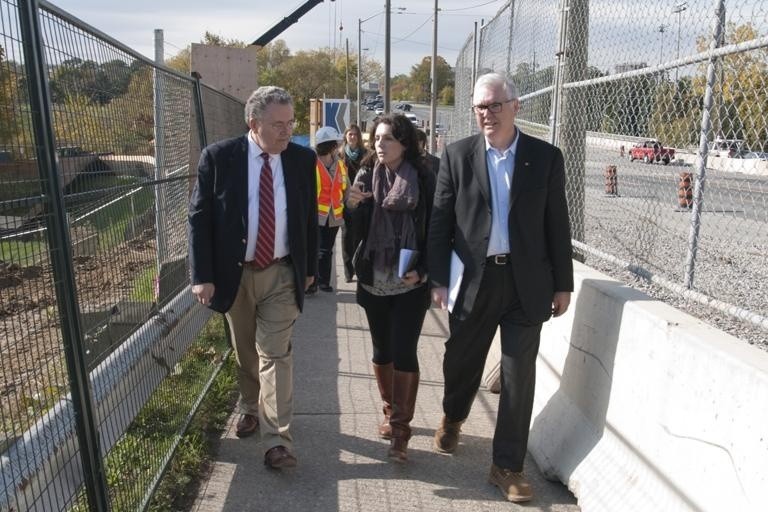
[187,85,321,470]
[344,112,436,466]
[304,95,441,296]
[425,73,574,503]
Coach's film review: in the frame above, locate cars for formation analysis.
[709,140,768,161]
[363,94,446,137]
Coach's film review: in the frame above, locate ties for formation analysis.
[254,152,275,270]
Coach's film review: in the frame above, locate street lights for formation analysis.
[673,2,688,94]
[357,7,406,132]
[658,24,667,66]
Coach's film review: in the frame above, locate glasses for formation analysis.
[470,98,514,116]
[261,119,294,130]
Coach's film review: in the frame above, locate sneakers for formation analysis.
[434,415,467,453]
[488,462,534,502]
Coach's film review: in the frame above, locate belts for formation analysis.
[486,254,511,266]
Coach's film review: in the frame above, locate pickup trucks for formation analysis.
[630,139,675,166]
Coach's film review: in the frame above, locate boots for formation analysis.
[371,358,394,439]
[387,364,420,465]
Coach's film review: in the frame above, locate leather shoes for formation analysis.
[235,413,259,437]
[319,283,333,292]
[264,446,297,468]
[304,278,318,295]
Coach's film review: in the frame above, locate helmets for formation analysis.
[315,126,344,147]
